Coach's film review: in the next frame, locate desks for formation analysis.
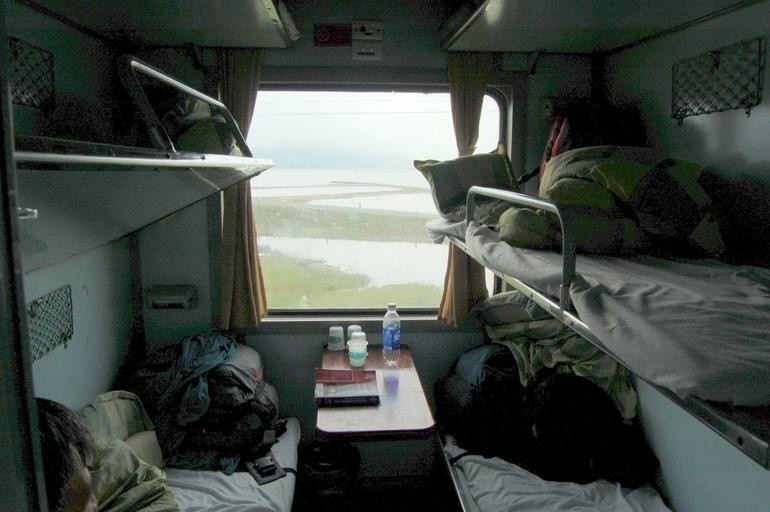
[315,344,437,441]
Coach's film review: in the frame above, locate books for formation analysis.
[314,368,377,387]
[314,381,380,408]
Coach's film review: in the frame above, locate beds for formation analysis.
[439,1,757,57]
[439,433,675,511]
[18,1,300,49]
[10,54,273,277]
[136,417,301,512]
[423,182,770,469]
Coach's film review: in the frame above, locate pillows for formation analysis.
[468,290,555,325]
[413,142,521,221]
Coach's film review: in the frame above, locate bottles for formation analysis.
[382,303,401,368]
[383,368,399,397]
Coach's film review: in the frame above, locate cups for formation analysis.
[351,331,366,345]
[326,326,346,351]
[346,340,369,368]
[347,325,361,349]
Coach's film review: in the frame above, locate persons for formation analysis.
[35,398,101,511]
